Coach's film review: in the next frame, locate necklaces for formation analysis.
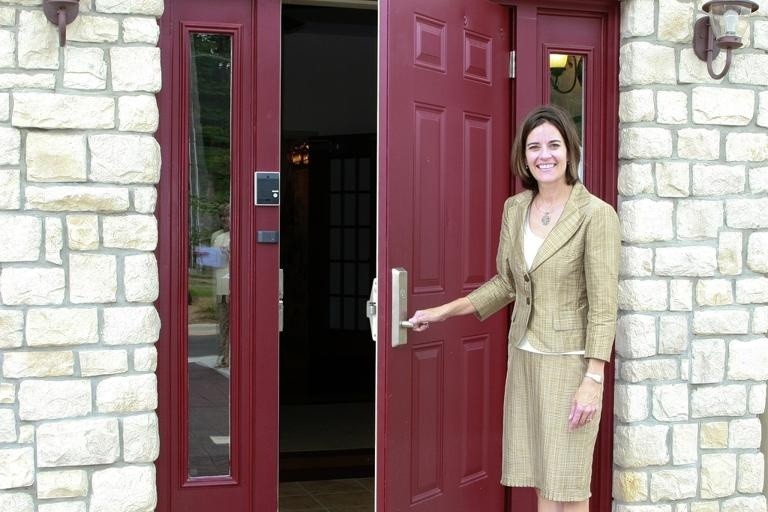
[534,187,570,226]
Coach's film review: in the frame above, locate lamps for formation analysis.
[694,0,759,79]
[550,52,577,94]
[286,142,311,187]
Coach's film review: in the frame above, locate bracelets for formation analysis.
[585,371,604,384]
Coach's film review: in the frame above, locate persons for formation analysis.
[406,101,622,511]
[210,202,229,369]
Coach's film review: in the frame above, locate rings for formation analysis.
[586,416,592,422]
